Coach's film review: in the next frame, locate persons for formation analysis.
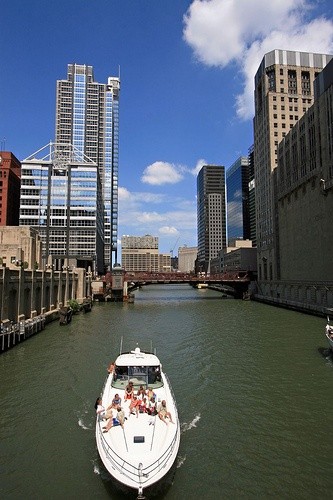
[157,400,174,424]
[124,381,134,400]
[147,397,157,415]
[140,397,147,413]
[94,397,113,419]
[127,396,139,418]
[111,393,122,412]
[136,385,145,397]
[102,407,125,433]
[146,387,157,396]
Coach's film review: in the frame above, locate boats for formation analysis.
[95,335,181,497]
[325,316,333,350]
